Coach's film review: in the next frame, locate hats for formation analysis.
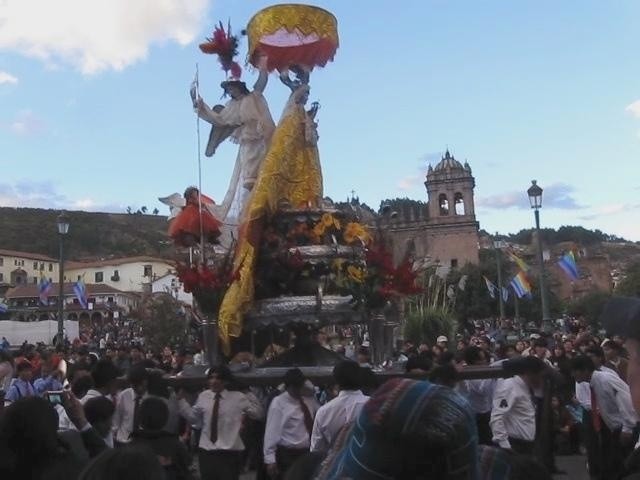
[530,333,541,339]
[437,335,448,342]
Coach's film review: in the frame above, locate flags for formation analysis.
[72,278,88,311]
[502,246,529,273]
[37,277,53,301]
[482,276,494,299]
[551,252,581,281]
[502,287,509,302]
[509,273,533,298]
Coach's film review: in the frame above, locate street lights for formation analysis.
[492,231,506,320]
[508,245,521,326]
[527,181,552,333]
[55,209,72,345]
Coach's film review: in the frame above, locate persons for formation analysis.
[13,309,60,321]
[125,398,191,476]
[69,317,176,391]
[82,394,115,449]
[190,365,263,479]
[262,369,322,480]
[172,187,218,253]
[1,340,70,406]
[109,365,191,448]
[189,54,276,250]
[1,390,107,478]
[309,362,372,479]
[240,84,321,210]
[382,314,640,479]
[79,447,168,477]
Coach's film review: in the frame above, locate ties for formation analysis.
[302,399,314,438]
[133,395,142,434]
[211,395,219,443]
[24,383,29,394]
[591,385,600,432]
